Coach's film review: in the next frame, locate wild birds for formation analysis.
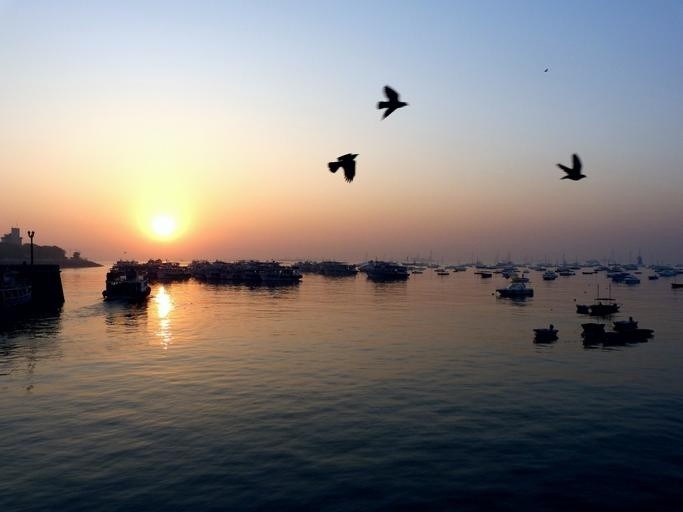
[376,84,409,117]
[327,153,360,184]
[555,151,588,182]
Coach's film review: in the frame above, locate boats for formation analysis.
[1,269,33,306]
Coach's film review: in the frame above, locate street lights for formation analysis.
[28,230,35,264]
